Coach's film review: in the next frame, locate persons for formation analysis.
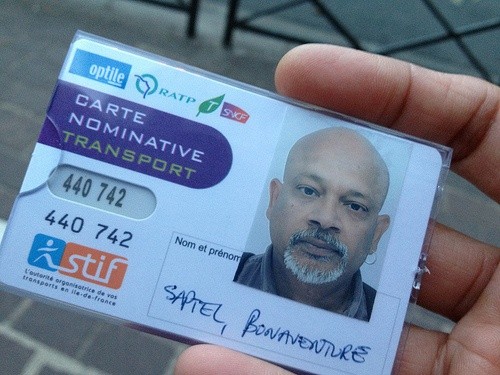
[173,42,500,375]
[233,125,392,321]
